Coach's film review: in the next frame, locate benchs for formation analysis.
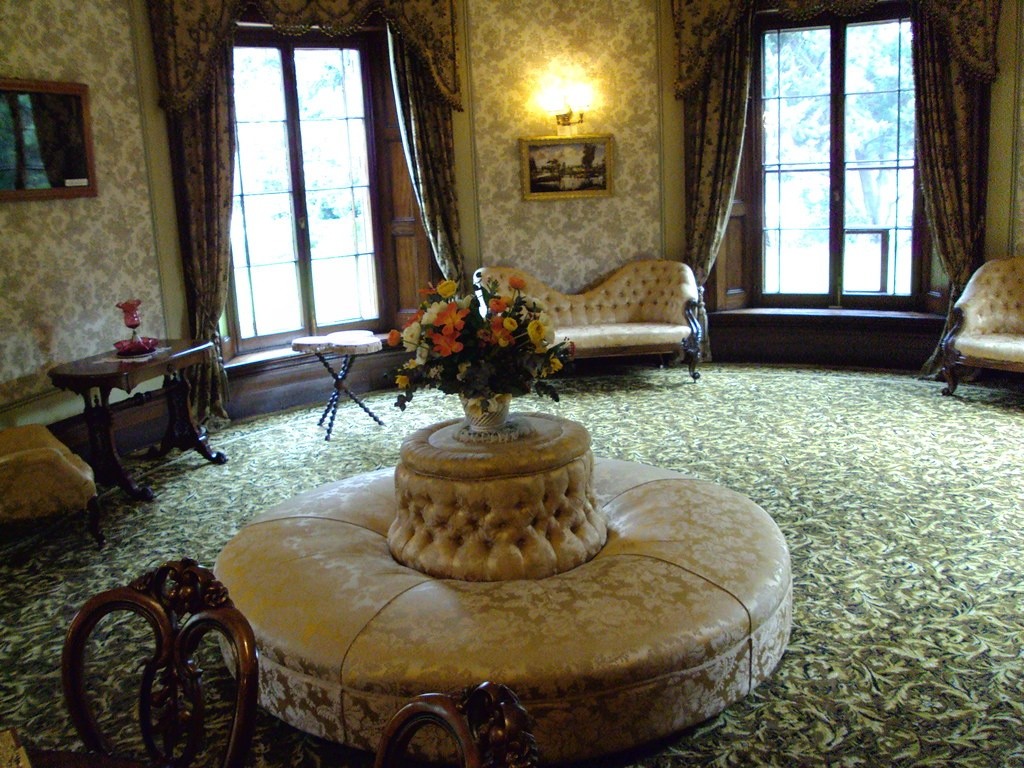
[211,454,796,764]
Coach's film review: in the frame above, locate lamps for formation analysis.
[529,62,595,126]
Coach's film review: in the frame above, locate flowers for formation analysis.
[115,297,144,328]
[387,274,578,410]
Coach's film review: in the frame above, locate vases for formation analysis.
[112,329,160,354]
[454,392,516,434]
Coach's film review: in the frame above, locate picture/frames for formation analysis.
[518,132,615,201]
[0,77,98,201]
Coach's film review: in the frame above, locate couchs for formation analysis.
[0,423,105,551]
[942,255,1024,397]
[475,259,702,383]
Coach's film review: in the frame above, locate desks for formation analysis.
[292,330,386,441]
[47,339,228,550]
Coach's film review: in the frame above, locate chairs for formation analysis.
[61,557,260,768]
